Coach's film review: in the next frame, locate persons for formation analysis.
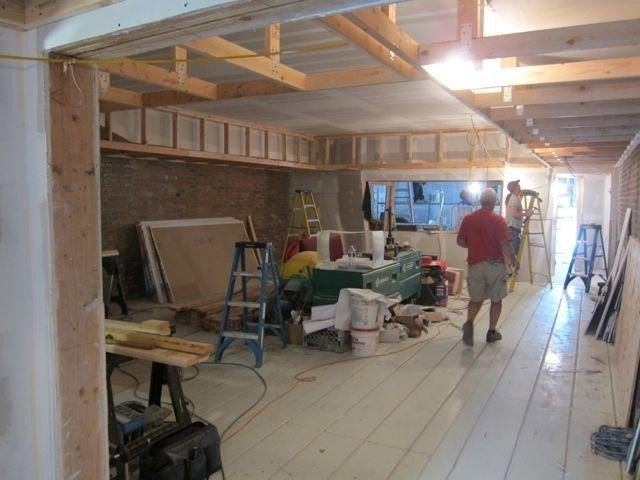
[454,188,521,346]
[458,190,474,206]
[504,179,534,256]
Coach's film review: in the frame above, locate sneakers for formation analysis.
[486,330,502,342]
[462,321,473,346]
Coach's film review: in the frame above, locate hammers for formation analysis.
[524,207,540,223]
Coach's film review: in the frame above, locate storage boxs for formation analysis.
[285,320,303,346]
[445,270,460,294]
[446,266,466,293]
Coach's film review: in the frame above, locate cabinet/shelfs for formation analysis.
[311,247,422,314]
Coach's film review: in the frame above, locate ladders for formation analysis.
[395,181,414,223]
[563,224,608,293]
[279,190,322,276]
[215,241,287,369]
[509,192,552,293]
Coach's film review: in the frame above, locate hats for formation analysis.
[507,179,520,193]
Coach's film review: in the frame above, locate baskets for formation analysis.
[302,320,351,353]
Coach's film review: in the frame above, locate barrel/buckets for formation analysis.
[350,326,380,358]
[349,297,380,330]
